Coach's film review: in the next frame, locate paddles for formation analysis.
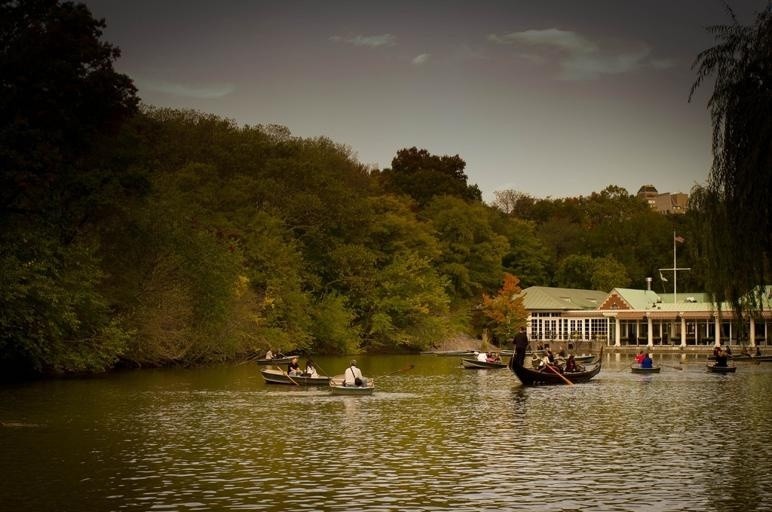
[373,364,414,381]
[658,364,682,371]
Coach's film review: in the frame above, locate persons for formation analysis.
[713,343,761,366]
[485,351,497,362]
[532,343,577,373]
[344,358,365,386]
[288,357,302,376]
[642,352,653,368]
[305,359,321,376]
[634,349,645,368]
[265,346,274,360]
[512,325,529,365]
[274,348,286,359]
[494,351,503,363]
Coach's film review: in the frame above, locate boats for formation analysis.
[260,368,335,385]
[461,358,507,369]
[507,355,601,385]
[329,374,375,395]
[631,366,661,373]
[705,353,772,373]
[256,355,300,365]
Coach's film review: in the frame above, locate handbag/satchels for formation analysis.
[355,377,362,386]
